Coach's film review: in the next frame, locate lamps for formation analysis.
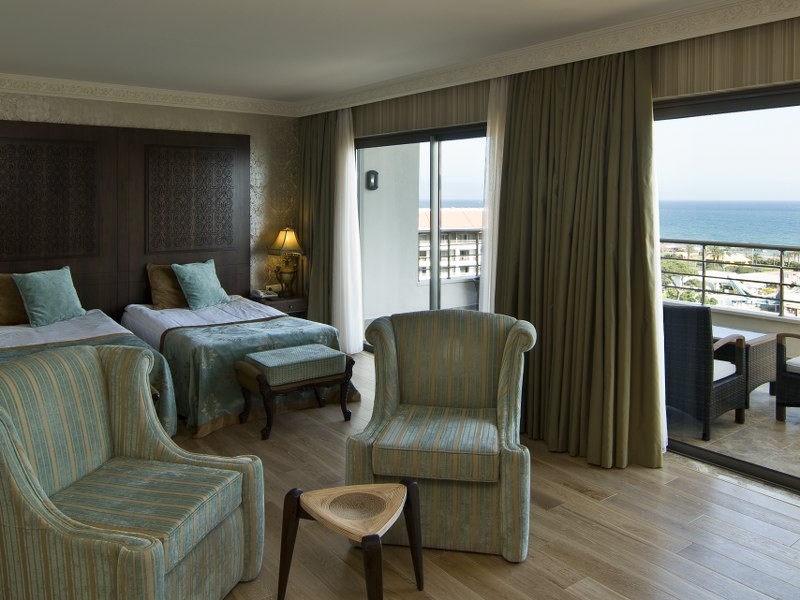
[367,170,378,190]
[267,228,301,298]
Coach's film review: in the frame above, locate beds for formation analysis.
[123,295,360,438]
[0,308,178,439]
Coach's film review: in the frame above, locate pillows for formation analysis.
[172,259,229,309]
[11,266,87,330]
[147,263,188,310]
[0,274,30,326]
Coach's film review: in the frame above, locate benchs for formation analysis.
[238,344,357,440]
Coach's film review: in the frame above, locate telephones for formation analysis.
[251,289,278,298]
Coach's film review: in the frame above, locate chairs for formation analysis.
[764,334,800,421]
[663,302,750,442]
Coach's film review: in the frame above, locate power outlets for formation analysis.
[266,284,281,292]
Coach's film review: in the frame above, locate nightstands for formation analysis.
[261,295,307,319]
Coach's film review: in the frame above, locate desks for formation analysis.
[276,481,424,600]
[710,325,778,409]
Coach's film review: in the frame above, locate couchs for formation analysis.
[0,346,265,600]
[346,309,539,562]
